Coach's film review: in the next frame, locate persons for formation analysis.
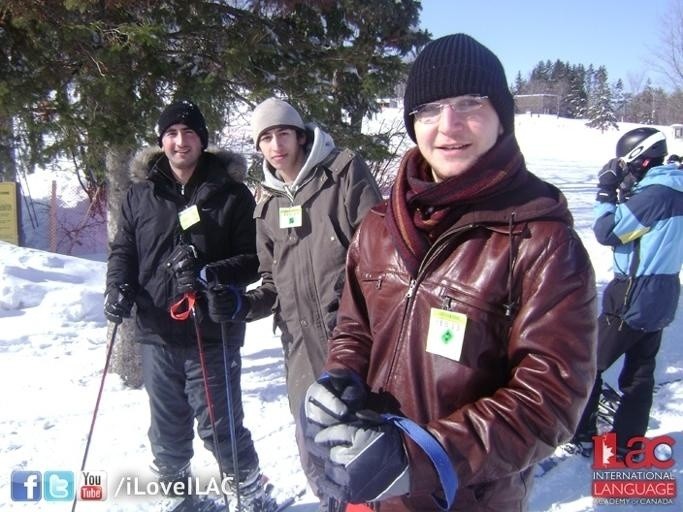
[103,99,258,512]
[206,95,388,512]
[302,32,599,512]
[559,128,683,463]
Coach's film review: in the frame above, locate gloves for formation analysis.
[300,373,412,503]
[175,260,250,323]
[595,158,637,203]
[103,284,132,323]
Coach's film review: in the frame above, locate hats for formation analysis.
[158,100,208,149]
[404,33,514,144]
[250,97,307,151]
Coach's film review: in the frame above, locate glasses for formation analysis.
[408,95,492,123]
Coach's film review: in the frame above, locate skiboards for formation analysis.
[532,382,650,485]
[165,479,305,512]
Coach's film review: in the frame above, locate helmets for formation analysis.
[616,127,668,169]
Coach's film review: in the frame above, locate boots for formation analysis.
[224,468,279,512]
[149,460,203,512]
